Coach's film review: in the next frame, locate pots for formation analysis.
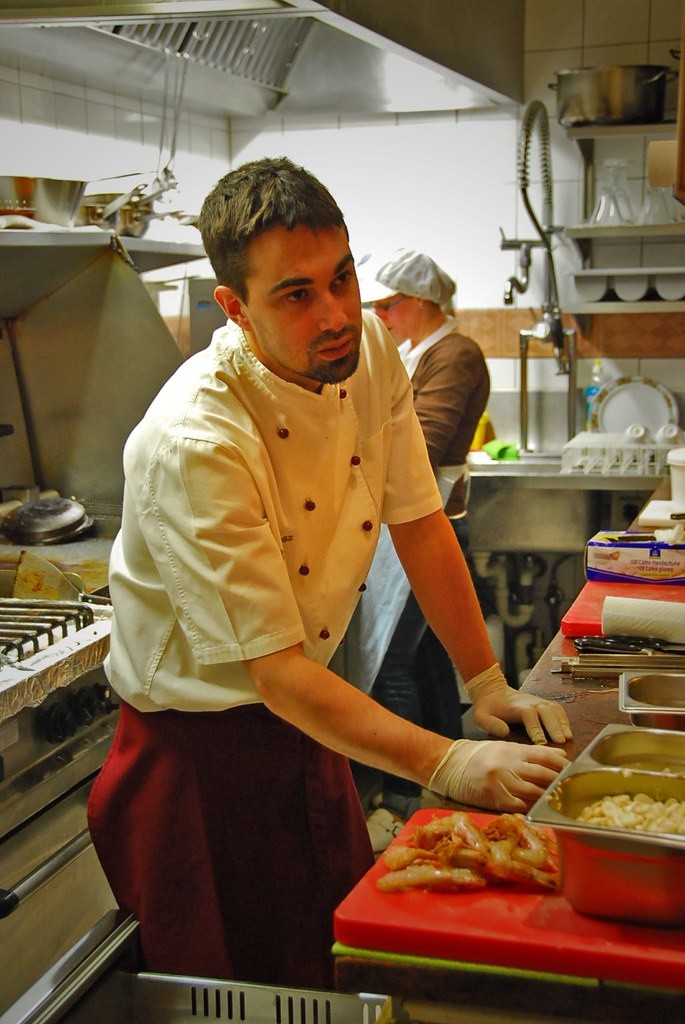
[74,167,184,238]
[548,64,678,127]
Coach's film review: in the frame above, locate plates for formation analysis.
[587,375,678,446]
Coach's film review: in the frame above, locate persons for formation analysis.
[350,246,488,854]
[85,154,573,991]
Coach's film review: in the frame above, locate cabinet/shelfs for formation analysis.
[562,118,685,315]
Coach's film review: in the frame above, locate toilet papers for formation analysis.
[600,594,685,644]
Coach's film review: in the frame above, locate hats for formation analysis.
[357,247,456,305]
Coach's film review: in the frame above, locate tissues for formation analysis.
[582,522,684,586]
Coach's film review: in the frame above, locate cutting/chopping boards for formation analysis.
[560,579,685,638]
[331,806,685,990]
[637,499,685,527]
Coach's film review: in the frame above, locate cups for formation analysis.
[624,424,654,446]
[666,450,685,504]
[654,425,684,447]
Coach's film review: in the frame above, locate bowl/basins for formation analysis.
[0,175,88,227]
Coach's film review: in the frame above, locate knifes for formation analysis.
[574,633,685,656]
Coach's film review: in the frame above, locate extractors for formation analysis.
[0,0,527,122]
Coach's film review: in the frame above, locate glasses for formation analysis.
[371,296,408,312]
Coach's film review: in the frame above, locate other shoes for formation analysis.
[365,808,405,853]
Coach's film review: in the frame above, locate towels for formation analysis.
[480,438,521,463]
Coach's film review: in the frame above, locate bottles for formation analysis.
[583,359,605,419]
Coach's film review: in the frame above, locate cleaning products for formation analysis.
[580,355,612,436]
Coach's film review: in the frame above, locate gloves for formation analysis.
[463,663,573,746]
[428,738,572,814]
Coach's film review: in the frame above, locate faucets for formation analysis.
[494,227,552,306]
